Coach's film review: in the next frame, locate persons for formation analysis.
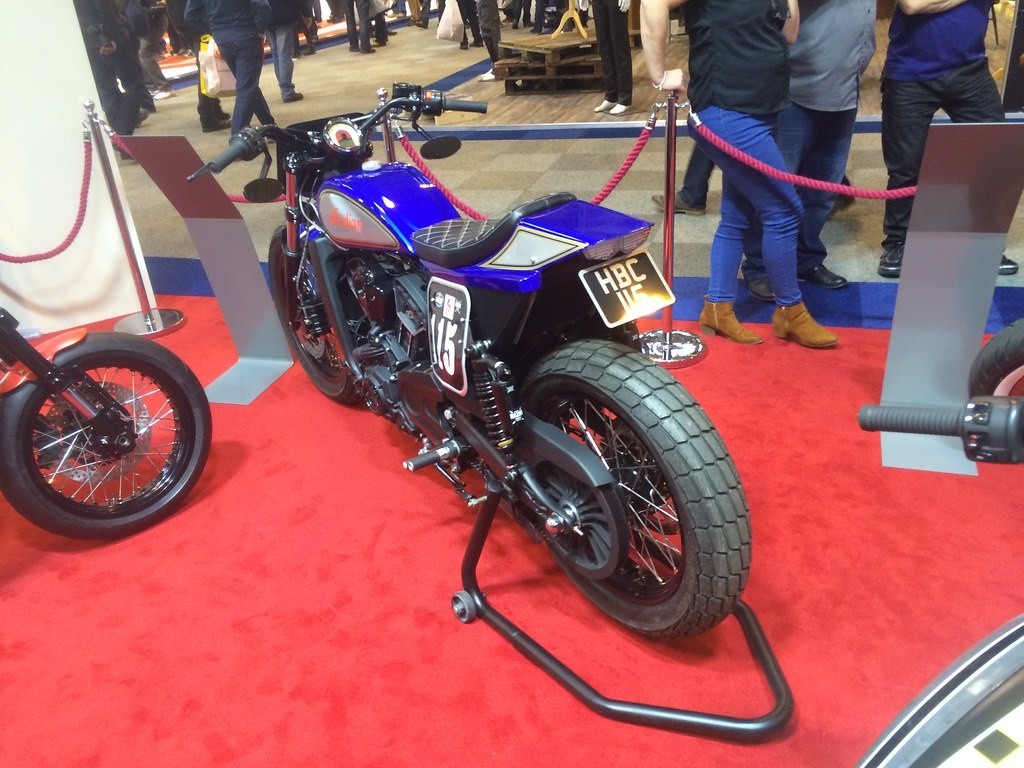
[579,0,635,115]
[639,0,1019,349]
[73,0,589,160]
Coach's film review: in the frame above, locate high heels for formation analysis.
[700,295,764,345]
[772,303,838,347]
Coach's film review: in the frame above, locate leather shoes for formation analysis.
[408,18,420,26]
[742,264,774,302]
[469,40,484,47]
[327,14,344,22]
[523,20,533,28]
[361,48,375,53]
[416,22,428,30]
[199,112,232,133]
[512,23,520,29]
[825,194,855,220]
[459,42,469,50]
[349,45,359,52]
[282,92,303,103]
[654,192,706,216]
[797,262,848,289]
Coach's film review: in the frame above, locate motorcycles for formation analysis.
[856,398,1023,768]
[184,80,753,640]
[0,308,211,542]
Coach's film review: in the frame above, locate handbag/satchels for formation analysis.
[199,35,236,97]
[347,0,397,26]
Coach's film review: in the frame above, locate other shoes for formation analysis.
[371,38,388,47]
[502,17,513,23]
[387,30,398,36]
[312,34,318,40]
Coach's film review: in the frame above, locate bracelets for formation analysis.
[654,70,667,89]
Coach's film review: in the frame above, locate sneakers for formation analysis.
[149,88,175,100]
[303,48,316,55]
[293,52,302,58]
[877,235,902,278]
[387,11,405,18]
[997,254,1019,275]
